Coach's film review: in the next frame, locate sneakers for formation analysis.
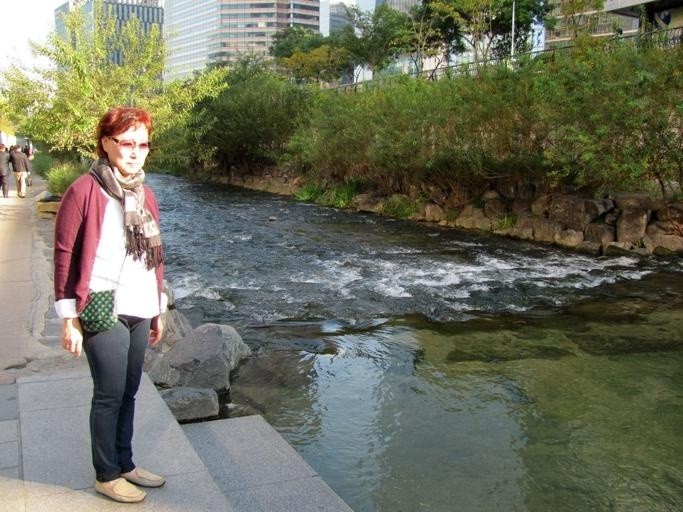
[121,466,166,488]
[94,477,146,503]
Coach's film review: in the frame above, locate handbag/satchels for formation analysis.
[77,288,118,333]
[25,175,34,186]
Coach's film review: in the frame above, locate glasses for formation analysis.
[110,136,150,151]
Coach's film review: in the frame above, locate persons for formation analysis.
[9,144,31,198]
[50,105,171,504]
[0,143,10,198]
[4,145,32,163]
